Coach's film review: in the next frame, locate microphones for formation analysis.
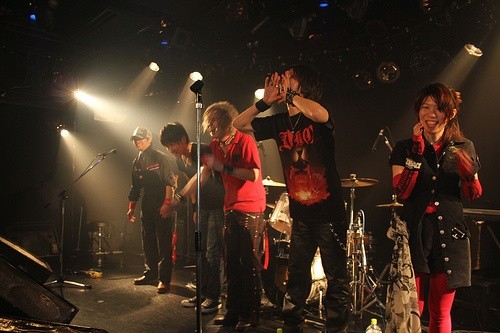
[98,149,118,156]
[355,211,361,222]
[371,128,386,151]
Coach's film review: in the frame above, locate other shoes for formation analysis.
[234,315,253,331]
[157,281,169,293]
[214,313,233,324]
[134,276,158,284]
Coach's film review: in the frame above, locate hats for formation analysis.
[130,127,152,140]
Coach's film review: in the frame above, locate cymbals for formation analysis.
[261,178,287,187]
[339,178,378,188]
[375,201,404,207]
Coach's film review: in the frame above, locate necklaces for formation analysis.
[289,113,301,135]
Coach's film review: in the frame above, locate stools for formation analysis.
[89,221,108,272]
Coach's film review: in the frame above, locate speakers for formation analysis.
[0,221,79,324]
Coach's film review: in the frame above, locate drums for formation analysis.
[345,229,374,254]
[259,220,328,308]
[269,191,293,235]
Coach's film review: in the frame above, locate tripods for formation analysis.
[42,156,105,300]
[347,181,387,319]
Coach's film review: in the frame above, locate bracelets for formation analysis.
[129,203,135,209]
[164,199,171,205]
[255,99,271,112]
[287,91,300,104]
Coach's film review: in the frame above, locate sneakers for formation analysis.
[181,295,206,306]
[195,297,223,313]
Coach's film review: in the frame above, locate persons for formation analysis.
[233,64,351,333]
[388,82,482,333]
[202,102,266,331]
[127,126,175,293]
[160,123,225,313]
